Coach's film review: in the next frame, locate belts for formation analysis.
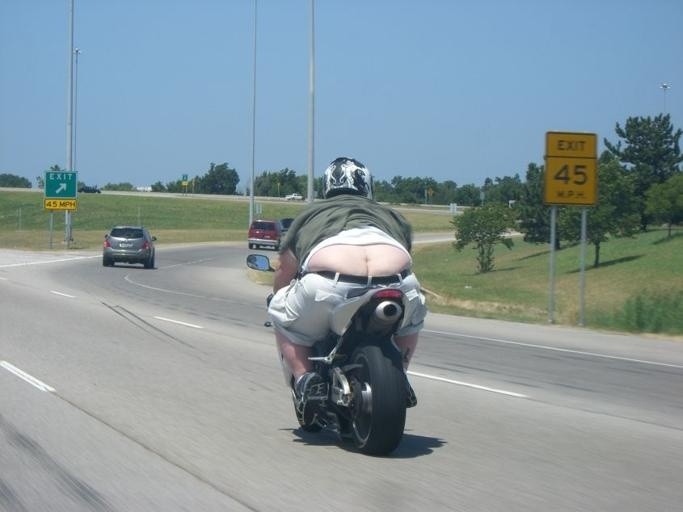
[303,270,411,289]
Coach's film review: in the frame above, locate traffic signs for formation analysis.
[44,198,76,209]
[546,130,598,204]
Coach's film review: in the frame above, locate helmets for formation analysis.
[321,157,374,199]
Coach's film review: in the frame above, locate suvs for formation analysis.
[247,219,281,250]
[101,224,157,268]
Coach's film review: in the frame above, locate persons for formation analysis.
[267,157,427,432]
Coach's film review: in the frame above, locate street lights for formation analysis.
[659,81,670,115]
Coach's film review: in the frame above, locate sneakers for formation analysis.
[292,372,329,432]
[404,372,417,409]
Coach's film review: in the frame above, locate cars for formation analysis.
[79,185,101,194]
[279,218,294,234]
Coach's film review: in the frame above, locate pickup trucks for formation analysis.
[285,193,305,200]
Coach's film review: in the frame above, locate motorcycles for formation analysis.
[245,251,417,456]
